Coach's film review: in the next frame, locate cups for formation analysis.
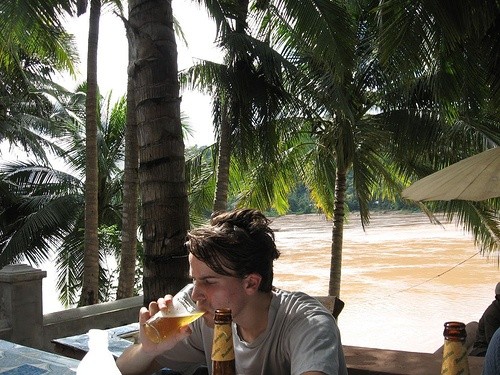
[143,283,208,344]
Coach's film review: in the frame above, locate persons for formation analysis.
[116,208,349,375]
[469,283,500,356]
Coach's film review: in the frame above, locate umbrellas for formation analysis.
[399,147,500,202]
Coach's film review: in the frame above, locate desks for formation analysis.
[0,339,82,375]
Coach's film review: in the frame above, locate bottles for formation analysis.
[440,322,470,375]
[74,328,122,374]
[211,308,238,375]
